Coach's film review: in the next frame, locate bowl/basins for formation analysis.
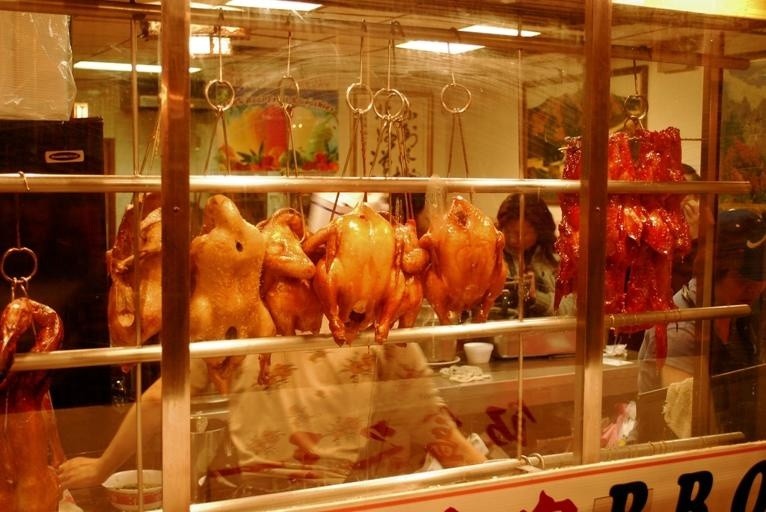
[101,469,162,512]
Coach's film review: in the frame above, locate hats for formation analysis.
[716,207,765,280]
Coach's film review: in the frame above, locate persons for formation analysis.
[497,191,576,314]
[56,312,491,498]
[635,207,765,446]
[671,165,701,294]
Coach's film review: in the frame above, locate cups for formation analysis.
[463,343,493,364]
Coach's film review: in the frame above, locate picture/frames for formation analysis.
[349,87,434,178]
[218,88,339,176]
[520,64,649,179]
